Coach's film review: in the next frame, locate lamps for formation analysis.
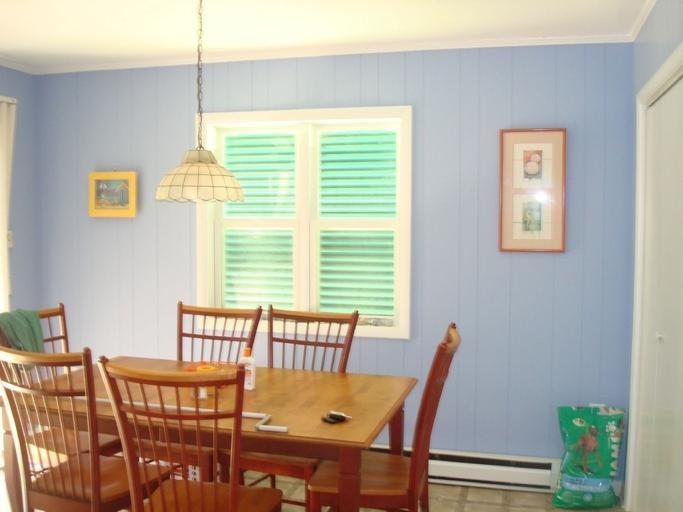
[154,0,246,202]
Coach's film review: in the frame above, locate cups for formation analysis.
[196,365,215,396]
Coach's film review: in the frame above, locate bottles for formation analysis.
[236,347,255,391]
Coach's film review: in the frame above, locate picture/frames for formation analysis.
[499,128,567,253]
[88,171,137,219]
